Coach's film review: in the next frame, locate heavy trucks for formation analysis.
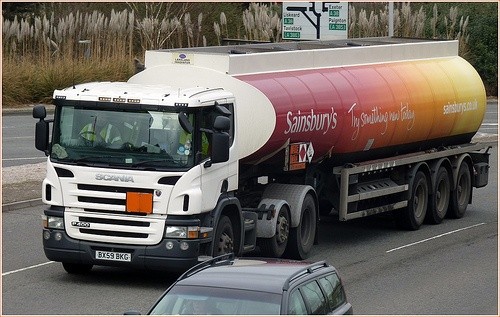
[32,36,492,279]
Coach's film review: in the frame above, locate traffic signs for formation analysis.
[282,1,349,40]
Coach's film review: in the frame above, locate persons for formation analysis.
[168,109,208,164]
[78,109,123,149]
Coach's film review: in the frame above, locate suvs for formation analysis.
[122,251,354,314]
